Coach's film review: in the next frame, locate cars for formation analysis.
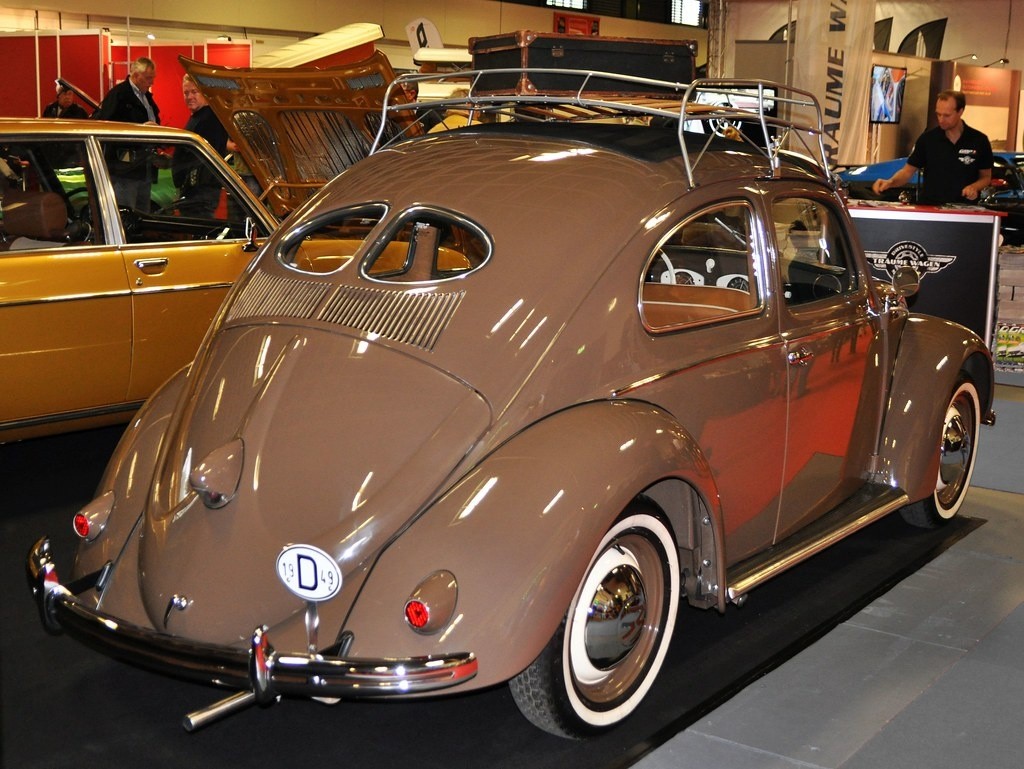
[0,117,471,453]
[898,154,1024,245]
[24,66,995,744]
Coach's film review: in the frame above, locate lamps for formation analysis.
[984,59,1010,67]
[947,54,979,62]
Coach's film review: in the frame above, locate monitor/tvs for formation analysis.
[869,63,908,123]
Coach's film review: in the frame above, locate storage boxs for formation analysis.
[467,29,699,103]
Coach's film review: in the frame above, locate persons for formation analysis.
[226,136,268,224]
[82,55,162,219]
[170,71,228,217]
[873,87,994,210]
[782,218,835,303]
[39,85,89,172]
[874,69,892,122]
[895,72,906,122]
[397,77,420,130]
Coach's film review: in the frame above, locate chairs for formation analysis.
[643,281,751,331]
[2,189,92,252]
